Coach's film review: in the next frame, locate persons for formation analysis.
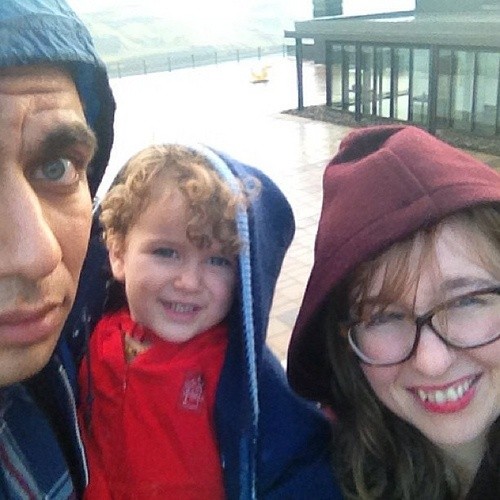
[260,124,500,500]
[57,142,332,500]
[1,0,118,500]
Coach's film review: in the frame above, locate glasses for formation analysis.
[341,283,500,368]
